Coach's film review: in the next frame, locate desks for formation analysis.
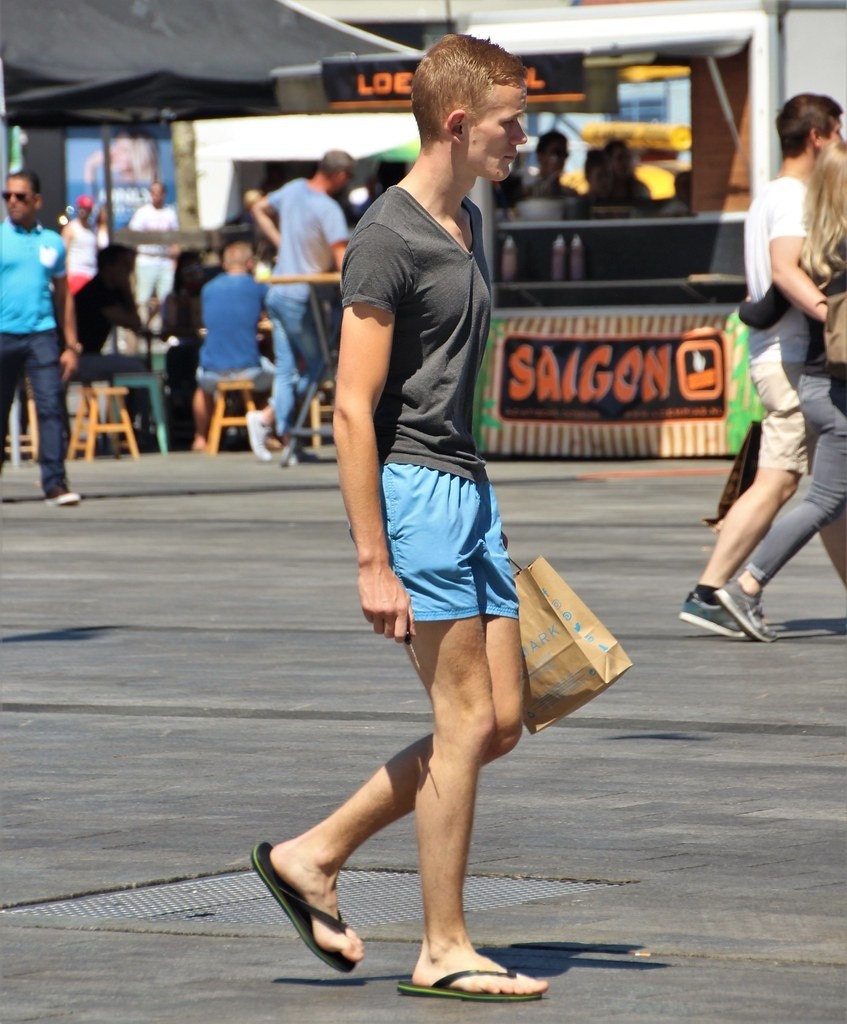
[270,271,342,466]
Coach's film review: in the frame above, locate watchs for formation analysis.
[66,342,83,355]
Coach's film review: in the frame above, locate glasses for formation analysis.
[1,191,27,202]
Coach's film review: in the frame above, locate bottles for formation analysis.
[501,236,515,282]
[570,235,583,280]
[554,235,565,278]
[146,288,159,321]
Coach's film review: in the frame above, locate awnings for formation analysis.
[464,14,753,155]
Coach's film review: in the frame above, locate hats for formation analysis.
[77,195,93,210]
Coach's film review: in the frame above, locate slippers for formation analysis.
[250,841,362,974]
[396,966,543,1002]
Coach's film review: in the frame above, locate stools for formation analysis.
[5,372,339,461]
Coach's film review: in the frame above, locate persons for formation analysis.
[84,130,157,197]
[160,250,205,428]
[62,195,109,295]
[252,34,547,1002]
[0,170,81,504]
[244,151,352,464]
[211,189,272,257]
[192,241,286,451]
[493,132,673,284]
[129,182,180,328]
[680,95,846,643]
[71,245,158,450]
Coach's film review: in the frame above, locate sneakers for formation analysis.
[676,592,749,639]
[716,580,775,644]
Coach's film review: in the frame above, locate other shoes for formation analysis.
[44,487,80,506]
[282,445,300,468]
[245,410,274,462]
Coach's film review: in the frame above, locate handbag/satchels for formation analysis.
[700,420,767,535]
[506,550,635,735]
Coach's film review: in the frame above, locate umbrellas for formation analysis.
[0,0,436,221]
